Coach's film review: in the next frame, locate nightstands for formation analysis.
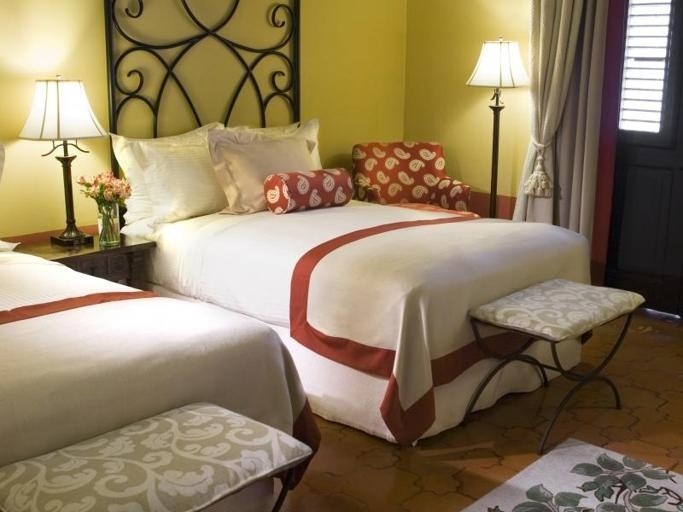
[0,225,157,286]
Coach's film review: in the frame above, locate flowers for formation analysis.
[76,170,132,242]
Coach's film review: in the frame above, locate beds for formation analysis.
[0,252,319,512]
[105,0,591,448]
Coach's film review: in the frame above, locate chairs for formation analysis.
[351,143,481,219]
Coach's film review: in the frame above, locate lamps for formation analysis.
[464,36,532,219]
[17,75,108,247]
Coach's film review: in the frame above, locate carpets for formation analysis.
[460,438,683,512]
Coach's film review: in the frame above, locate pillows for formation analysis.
[206,125,313,214]
[263,168,354,215]
[0,240,21,252]
[108,120,223,224]
[128,140,228,224]
[226,117,323,169]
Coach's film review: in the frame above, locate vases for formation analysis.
[96,203,119,247]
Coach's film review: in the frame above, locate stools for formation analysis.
[461,279,645,455]
[0,400,313,512]
